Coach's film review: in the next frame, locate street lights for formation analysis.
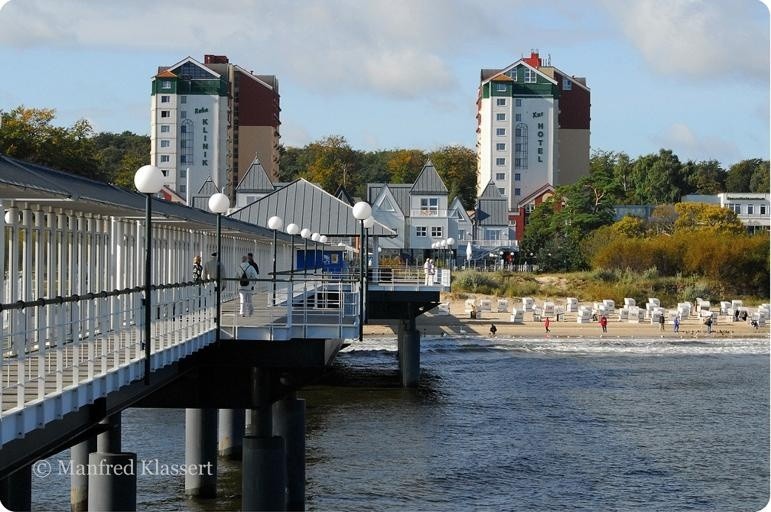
[135,165,165,386]
[3,210,21,347]
[209,193,230,348]
[499,251,504,269]
[432,238,455,270]
[301,228,313,291]
[353,201,374,341]
[320,235,328,263]
[287,223,299,304]
[529,253,534,272]
[268,216,284,307]
[511,252,516,272]
[312,232,321,273]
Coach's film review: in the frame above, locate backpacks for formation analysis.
[240,264,251,286]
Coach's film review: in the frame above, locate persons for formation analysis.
[424,258,430,285]
[601,315,607,332]
[490,324,497,335]
[658,313,665,331]
[236,255,257,317]
[544,316,550,333]
[200,253,226,323]
[674,318,679,332]
[192,257,203,281]
[248,253,259,275]
[708,310,758,334]
[429,260,435,284]
